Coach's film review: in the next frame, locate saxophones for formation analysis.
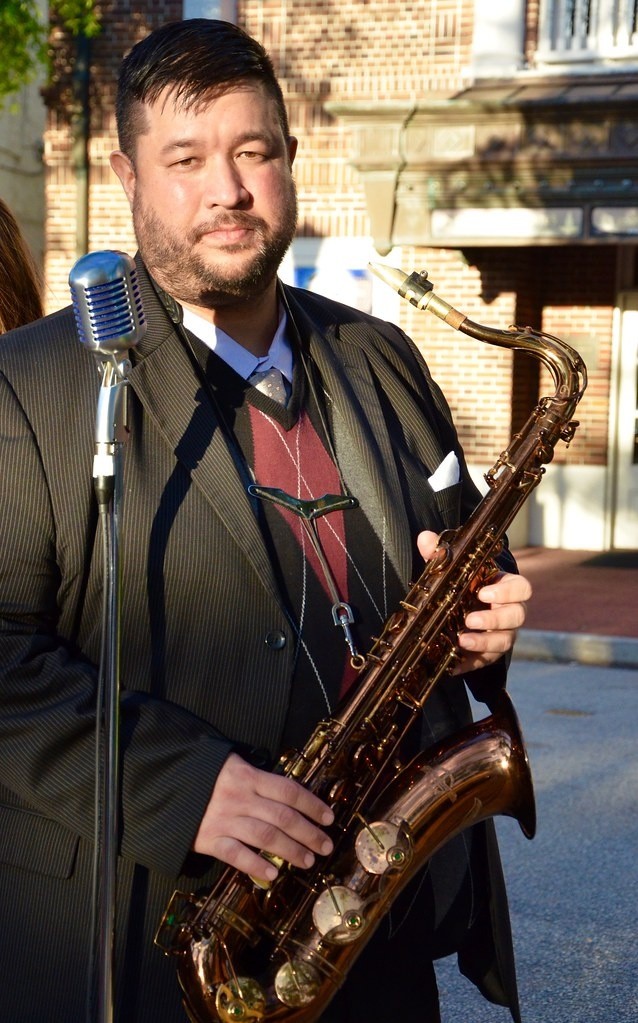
[142,257,594,1022]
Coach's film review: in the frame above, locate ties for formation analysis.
[248,369,286,406]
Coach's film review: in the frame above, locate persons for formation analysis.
[0,198,45,335]
[0,18,532,1023]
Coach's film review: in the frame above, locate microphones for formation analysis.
[68,250,147,515]
